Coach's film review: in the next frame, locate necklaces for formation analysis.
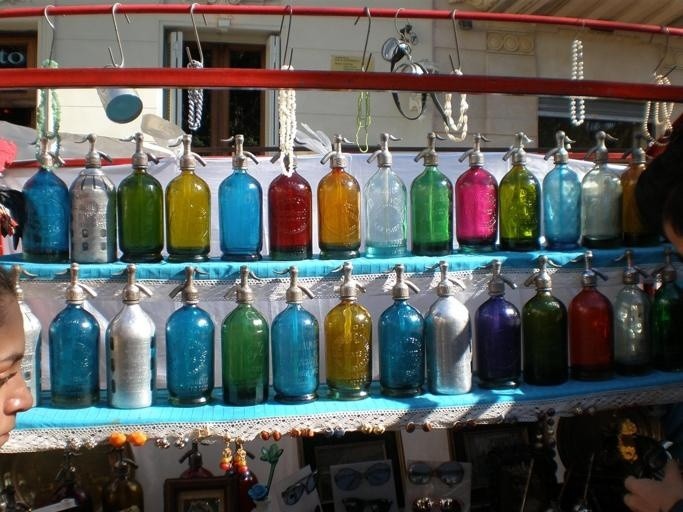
[32,58,64,172]
[569,40,586,127]
[273,65,299,179]
[641,73,675,147]
[441,69,470,144]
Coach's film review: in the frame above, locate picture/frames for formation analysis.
[161,476,240,512]
[297,429,411,512]
[446,420,559,512]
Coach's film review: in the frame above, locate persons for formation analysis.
[624,140,682,512]
[0,268,34,454]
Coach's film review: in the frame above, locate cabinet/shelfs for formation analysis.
[1,237,682,456]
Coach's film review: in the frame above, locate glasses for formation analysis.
[408,461,464,485]
[334,462,391,490]
[282,470,320,505]
[342,497,394,512]
[414,495,462,512]
